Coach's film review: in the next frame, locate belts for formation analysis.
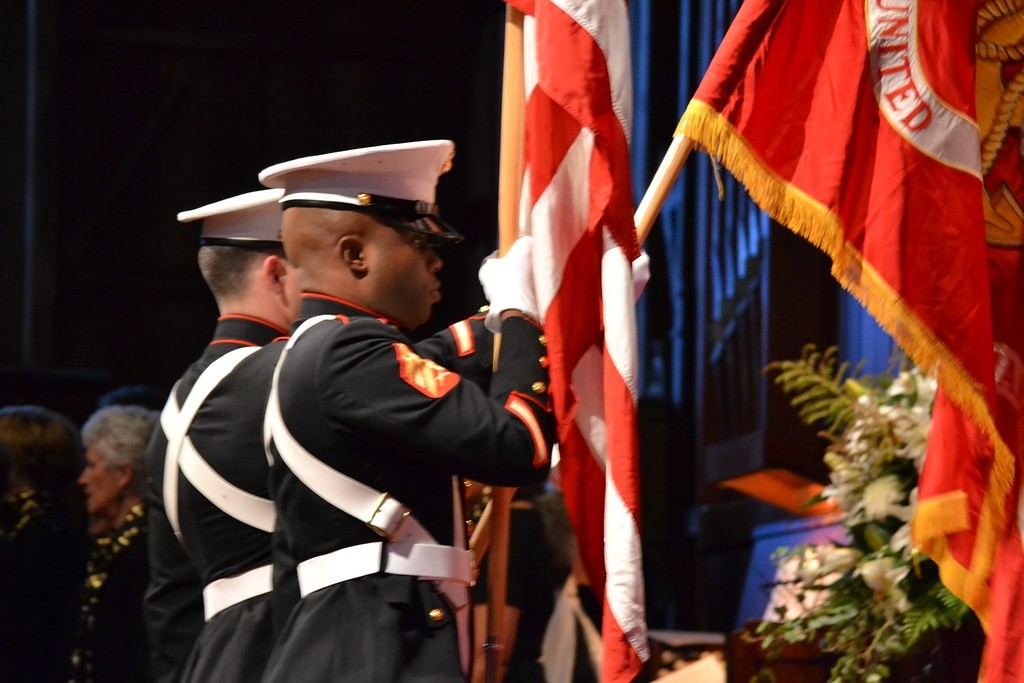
[296,545,477,600]
[203,563,274,622]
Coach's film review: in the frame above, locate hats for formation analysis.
[177,188,287,257]
[258,139,465,255]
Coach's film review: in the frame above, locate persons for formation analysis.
[0,405,79,683]
[66,403,158,683]
[137,184,648,682]
[258,138,559,682]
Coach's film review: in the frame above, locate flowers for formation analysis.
[748,344,970,683]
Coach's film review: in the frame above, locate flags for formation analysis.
[500,2,648,682]
[666,0,1024,682]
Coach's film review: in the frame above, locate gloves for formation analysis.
[478,235,539,334]
[632,248,651,304]
